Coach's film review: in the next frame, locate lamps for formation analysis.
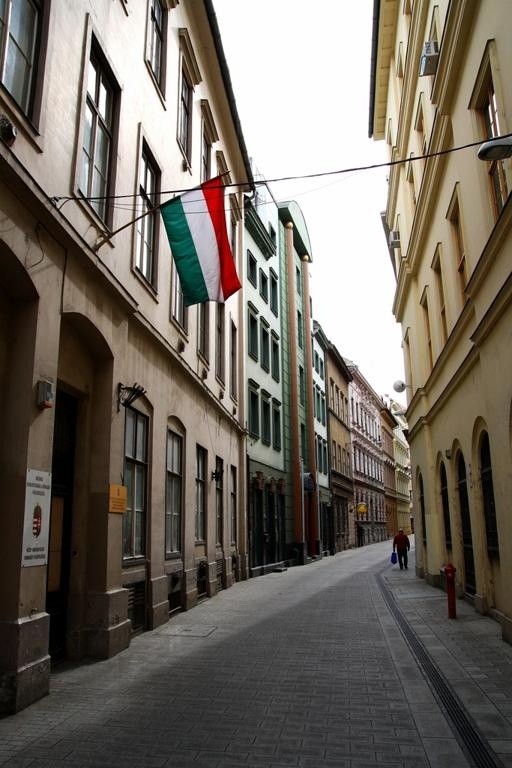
[392,379,411,393]
[119,380,146,412]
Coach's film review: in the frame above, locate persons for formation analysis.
[393,529,410,570]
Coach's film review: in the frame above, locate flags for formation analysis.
[159,176,242,308]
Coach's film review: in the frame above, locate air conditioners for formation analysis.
[388,231,399,248]
[419,40,438,77]
